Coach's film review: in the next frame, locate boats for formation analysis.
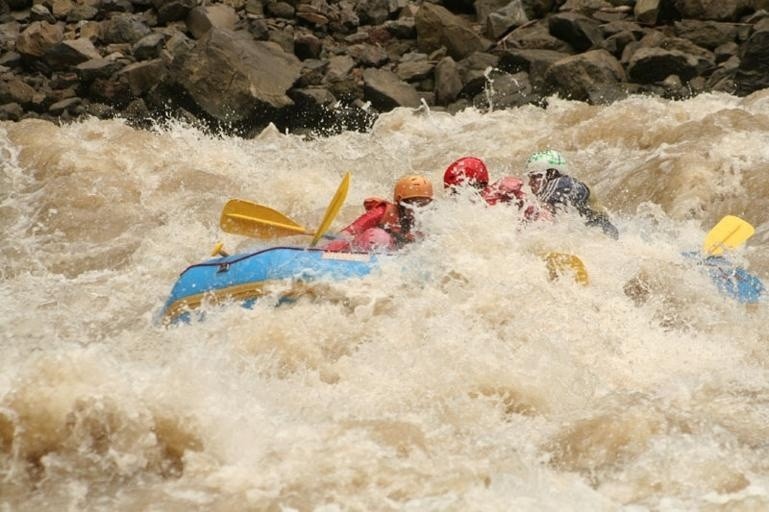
[162,241,761,329]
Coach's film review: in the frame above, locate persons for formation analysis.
[320,226,396,255]
[444,157,539,221]
[527,149,619,240]
[326,174,434,255]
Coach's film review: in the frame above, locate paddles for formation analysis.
[310,172,349,247]
[703,215,755,258]
[549,251,588,288]
[220,199,354,242]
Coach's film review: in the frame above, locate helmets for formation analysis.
[444,157,488,190]
[393,174,434,203]
[524,150,571,177]
